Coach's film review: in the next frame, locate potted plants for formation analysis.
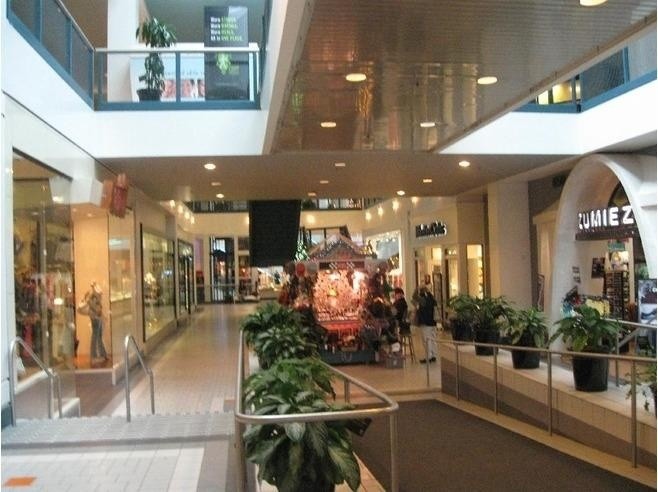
[545,302,627,393]
[131,13,178,101]
[445,295,476,345]
[623,351,657,418]
[453,296,518,357]
[488,302,549,369]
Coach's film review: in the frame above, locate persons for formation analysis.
[180,79,194,97]
[198,80,206,96]
[144,272,157,328]
[161,80,175,95]
[382,288,407,334]
[274,270,280,284]
[87,280,105,365]
[412,286,437,363]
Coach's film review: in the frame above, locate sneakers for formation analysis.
[419,359,427,363]
[429,356,436,363]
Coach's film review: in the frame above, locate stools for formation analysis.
[398,331,417,364]
[378,334,396,369]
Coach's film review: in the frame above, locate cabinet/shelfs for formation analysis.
[633,277,657,358]
[603,269,632,320]
